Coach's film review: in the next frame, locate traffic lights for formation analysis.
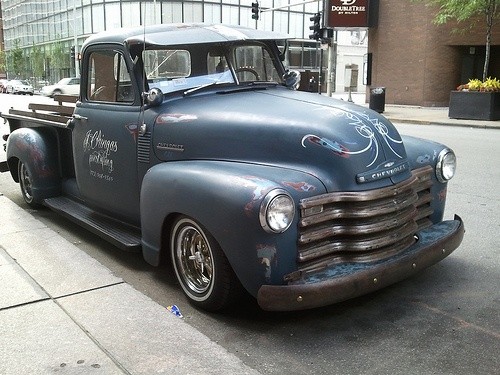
[322,38,331,45]
[309,12,323,40]
[70,45,75,58]
[251,2,259,20]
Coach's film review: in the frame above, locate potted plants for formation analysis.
[448,76,500,121]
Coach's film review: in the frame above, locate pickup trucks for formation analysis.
[0,22,465,316]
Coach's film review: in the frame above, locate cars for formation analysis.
[41,77,96,99]
[4,79,34,96]
[0,79,11,94]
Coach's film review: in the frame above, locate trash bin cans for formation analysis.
[369,87,386,113]
